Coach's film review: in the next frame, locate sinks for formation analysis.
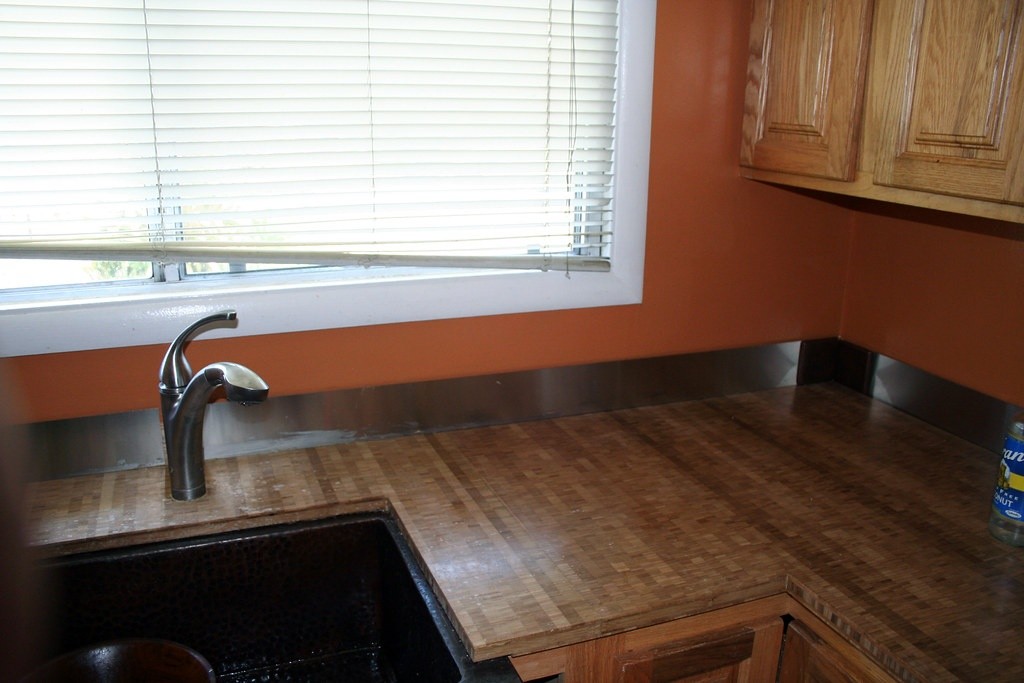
[13,495,474,683]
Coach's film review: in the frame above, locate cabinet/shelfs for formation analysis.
[600,615,783,682]
[776,619,864,682]
[737,0,1023,228]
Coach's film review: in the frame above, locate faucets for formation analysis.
[156,307,270,501]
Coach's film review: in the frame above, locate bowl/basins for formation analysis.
[17,640,218,683]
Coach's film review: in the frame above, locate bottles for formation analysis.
[987,409,1024,547]
